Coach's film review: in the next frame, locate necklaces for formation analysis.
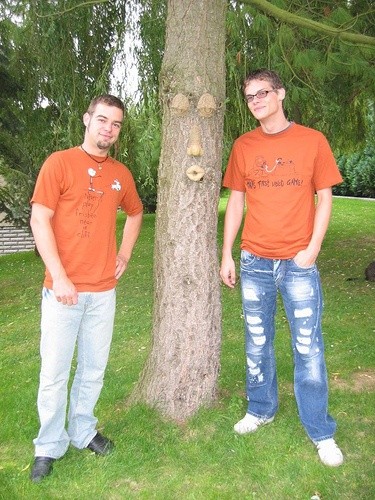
[81,144,109,171]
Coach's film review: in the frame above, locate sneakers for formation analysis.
[314,438,343,467]
[234,413,275,435]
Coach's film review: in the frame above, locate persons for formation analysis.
[29,94,144,483]
[217,68,345,467]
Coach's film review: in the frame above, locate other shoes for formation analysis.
[29,455,53,484]
[87,433,116,457]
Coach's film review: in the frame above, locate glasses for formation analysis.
[243,89,279,102]
